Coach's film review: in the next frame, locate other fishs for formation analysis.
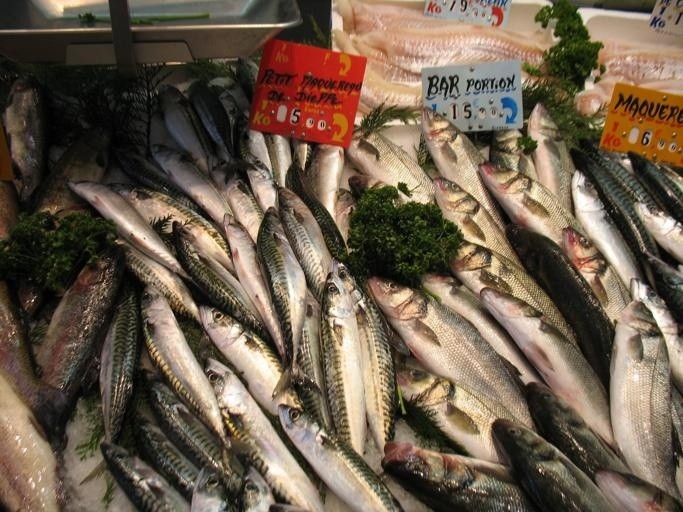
[0,52,683,512]
